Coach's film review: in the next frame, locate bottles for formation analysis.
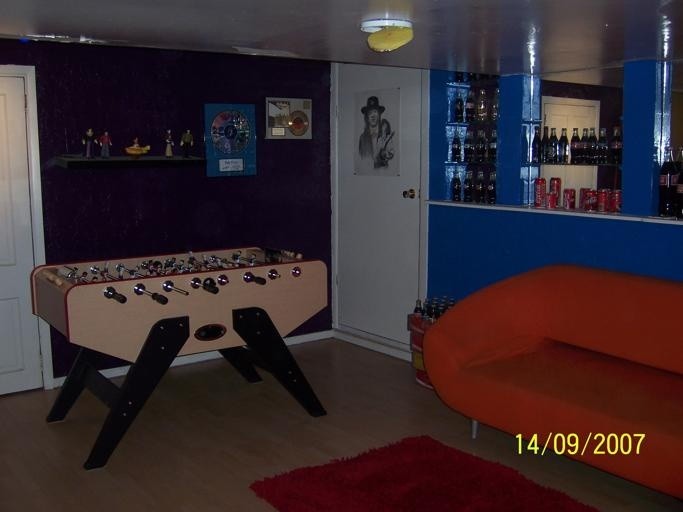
[658,147,679,215]
[675,148,683,219]
[530,123,621,164]
[448,71,497,206]
[412,296,456,323]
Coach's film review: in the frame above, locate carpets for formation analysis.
[247,430,603,511]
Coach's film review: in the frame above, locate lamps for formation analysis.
[358,18,414,54]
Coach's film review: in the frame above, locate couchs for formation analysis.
[422,258,683,498]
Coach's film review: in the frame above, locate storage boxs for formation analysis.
[404,312,440,390]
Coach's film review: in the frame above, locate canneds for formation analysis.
[534,177,622,215]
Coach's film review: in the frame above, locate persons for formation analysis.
[358,95,396,169]
[81,128,193,158]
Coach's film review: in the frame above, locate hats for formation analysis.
[361,96,385,114]
